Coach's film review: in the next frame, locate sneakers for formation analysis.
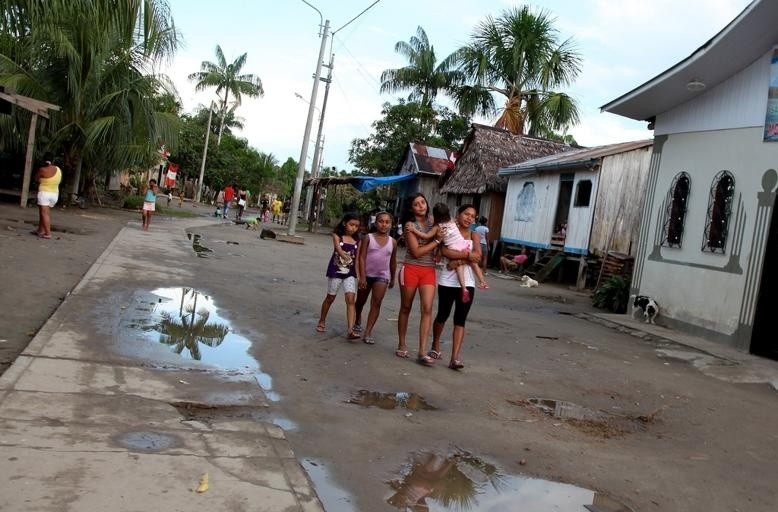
[479,283,489,290]
[463,289,469,302]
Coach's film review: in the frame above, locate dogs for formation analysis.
[519,274,539,288]
[630,294,659,325]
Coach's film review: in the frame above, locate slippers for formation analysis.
[347,324,374,344]
[396,348,408,357]
[450,359,463,369]
[429,350,441,358]
[416,355,434,365]
[31,230,51,238]
[317,323,326,333]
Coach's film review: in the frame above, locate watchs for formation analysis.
[435,240,440,244]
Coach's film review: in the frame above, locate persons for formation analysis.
[405,202,489,302]
[351,211,398,344]
[33,153,62,239]
[430,204,482,368]
[500,248,530,275]
[316,213,361,339]
[168,184,291,230]
[142,179,158,229]
[396,193,447,366]
[475,216,490,276]
